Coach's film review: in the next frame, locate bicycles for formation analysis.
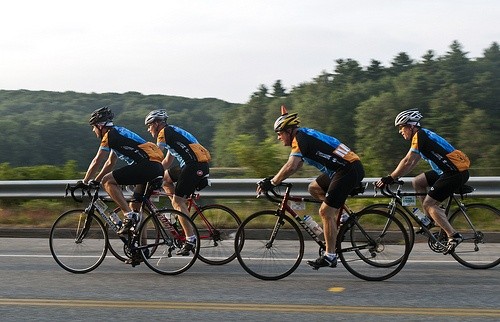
[102,172,244,265]
[234,180,410,282]
[349,177,500,270]
[49,175,200,276]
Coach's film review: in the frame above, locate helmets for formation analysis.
[273,112,300,131]
[145,109,168,125]
[89,107,112,125]
[395,109,423,127]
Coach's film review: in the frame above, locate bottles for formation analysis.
[412,207,431,226]
[109,212,123,229]
[304,214,324,237]
[161,206,177,225]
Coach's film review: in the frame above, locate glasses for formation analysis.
[277,130,286,136]
[147,124,154,129]
[399,124,405,130]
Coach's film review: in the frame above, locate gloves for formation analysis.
[76,181,88,190]
[88,179,100,189]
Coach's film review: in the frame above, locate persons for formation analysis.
[76,106,165,264]
[376,109,470,256]
[144,109,211,255]
[255,106,365,269]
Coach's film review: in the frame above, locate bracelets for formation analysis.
[269,179,277,187]
[82,180,88,185]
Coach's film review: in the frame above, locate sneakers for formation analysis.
[442,233,462,255]
[339,214,349,225]
[415,218,436,234]
[306,254,338,269]
[117,213,137,234]
[175,239,196,255]
[125,249,148,263]
